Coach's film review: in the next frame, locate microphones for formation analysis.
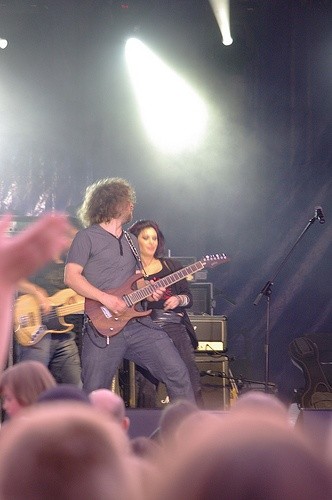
[315,207,325,224]
[200,370,214,376]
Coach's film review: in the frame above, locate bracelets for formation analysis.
[174,294,182,304]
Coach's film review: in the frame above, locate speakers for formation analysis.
[124,355,228,444]
[295,409,332,451]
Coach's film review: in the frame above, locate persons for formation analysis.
[127,219,199,409]
[0,213,70,374]
[0,353,332,500]
[64,178,199,418]
[16,217,83,390]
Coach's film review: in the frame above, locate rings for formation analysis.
[114,311,119,315]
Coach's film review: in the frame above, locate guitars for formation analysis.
[13,288,85,346]
[85,252,231,339]
